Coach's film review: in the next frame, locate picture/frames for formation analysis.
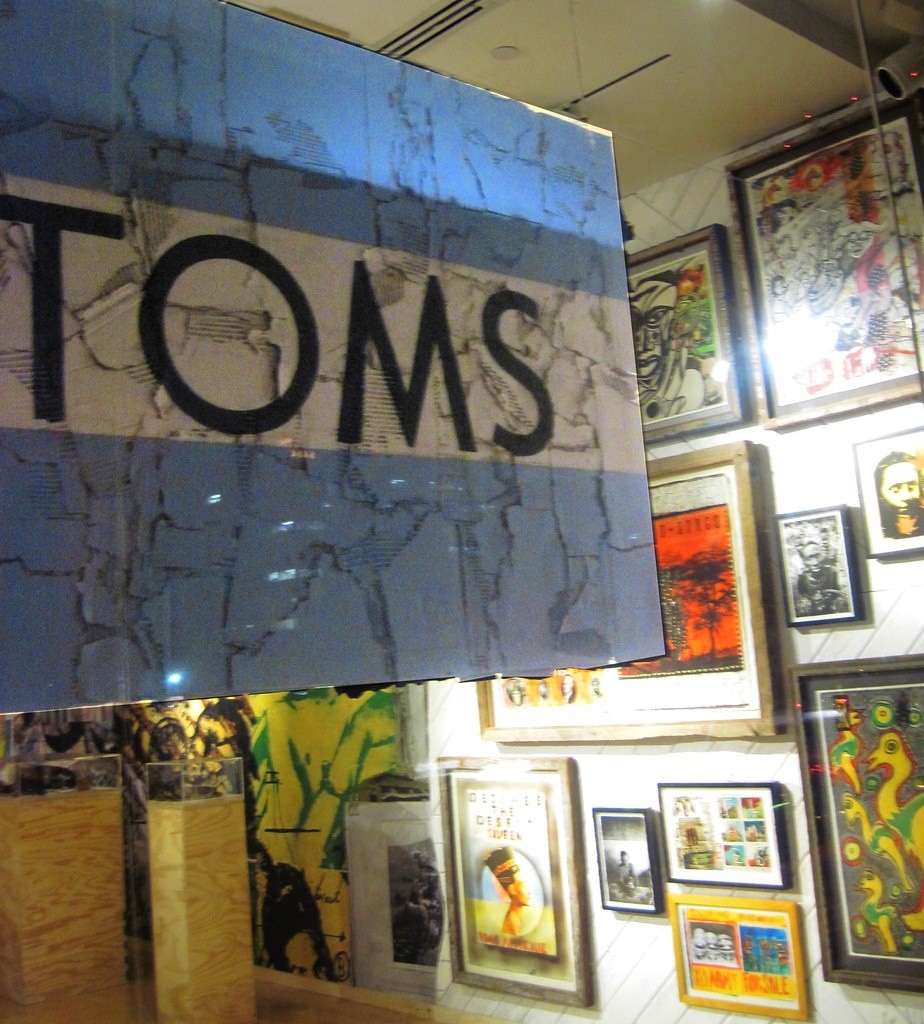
[788,652,924,990]
[772,505,864,625]
[473,438,780,742]
[664,893,812,1022]
[436,755,590,1009]
[343,800,435,995]
[853,425,924,559]
[591,807,663,915]
[723,88,923,429]
[657,778,794,891]
[623,221,751,442]
